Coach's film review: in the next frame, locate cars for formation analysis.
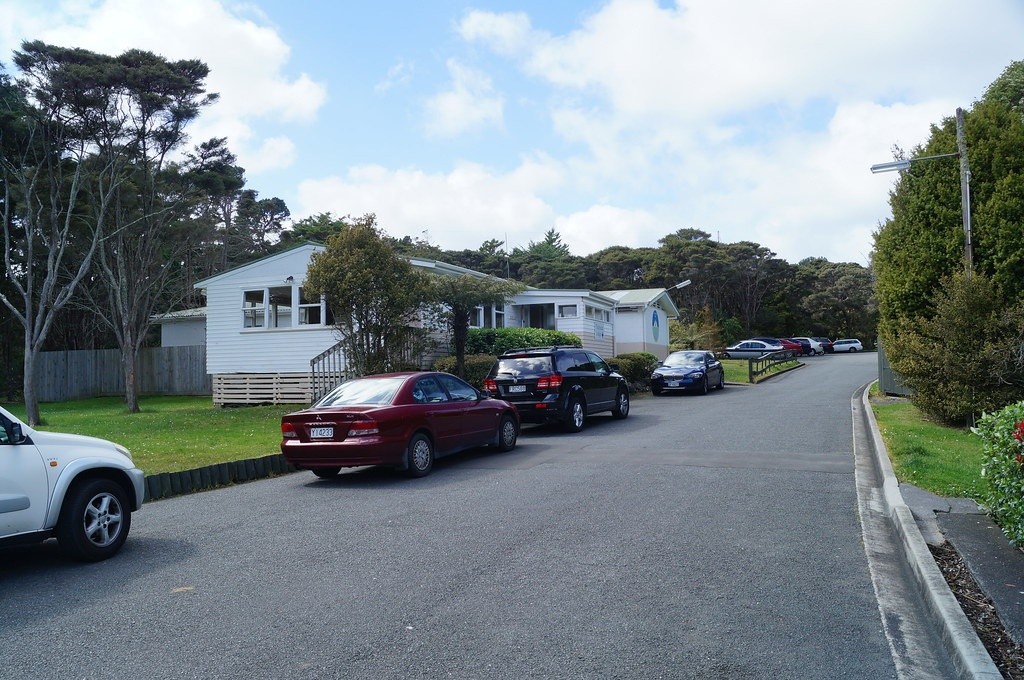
[650,350,725,397]
[280,369,520,481]
[721,340,785,362]
[751,337,822,358]
[0,408,145,564]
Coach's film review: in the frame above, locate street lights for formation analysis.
[871,107,976,277]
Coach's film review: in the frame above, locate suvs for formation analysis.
[810,337,833,356]
[484,343,631,432]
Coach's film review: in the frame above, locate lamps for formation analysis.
[283,274,294,283]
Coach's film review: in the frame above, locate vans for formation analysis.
[831,339,863,353]
